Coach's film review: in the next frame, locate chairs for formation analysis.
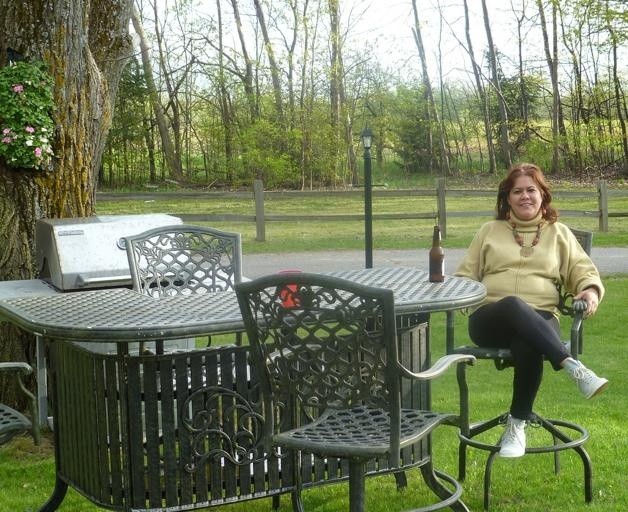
[446,226,591,511]
[125,226,242,295]
[0,360,42,448]
[234,272,479,512]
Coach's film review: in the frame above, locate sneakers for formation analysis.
[500,414,526,457]
[568,361,608,399]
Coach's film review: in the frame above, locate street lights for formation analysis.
[360,128,374,267]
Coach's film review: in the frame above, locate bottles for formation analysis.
[429,225,445,283]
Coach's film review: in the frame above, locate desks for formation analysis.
[0,267,487,512]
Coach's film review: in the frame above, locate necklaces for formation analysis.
[505,212,546,257]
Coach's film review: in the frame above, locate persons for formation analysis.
[452,162,610,458]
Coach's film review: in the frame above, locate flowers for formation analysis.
[0,61,57,172]
